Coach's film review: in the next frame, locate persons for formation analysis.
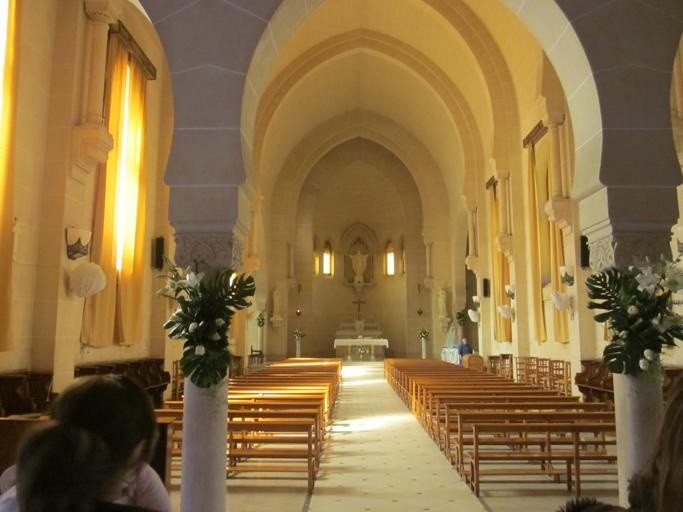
[457,337,474,368]
[0,374,172,512]
[554,373,683,512]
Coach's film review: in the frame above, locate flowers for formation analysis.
[587,251,681,376]
[417,327,429,339]
[294,327,304,339]
[156,252,257,391]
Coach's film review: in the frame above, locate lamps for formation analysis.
[65,225,92,259]
[503,284,514,299]
[558,266,573,286]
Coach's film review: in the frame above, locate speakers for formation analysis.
[155,236,163,269]
[580,236,590,267]
[484,279,489,296]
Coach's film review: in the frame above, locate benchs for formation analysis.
[381,354,616,499]
[151,352,342,494]
